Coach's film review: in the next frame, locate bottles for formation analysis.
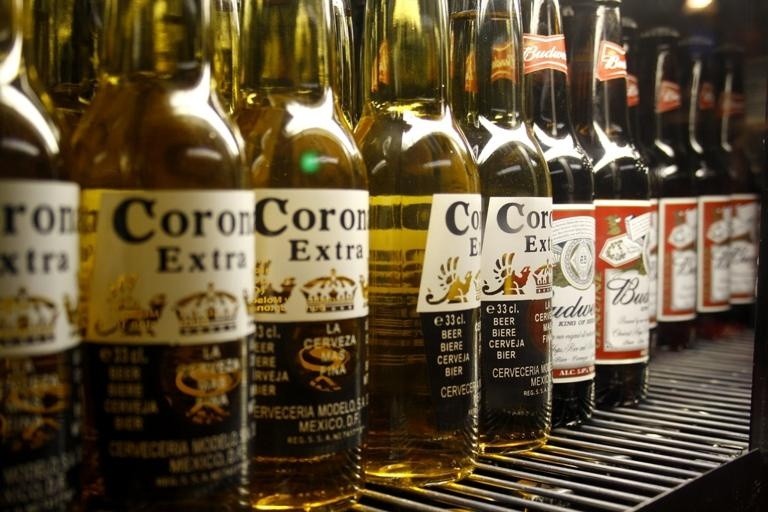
[0,0,767,511]
